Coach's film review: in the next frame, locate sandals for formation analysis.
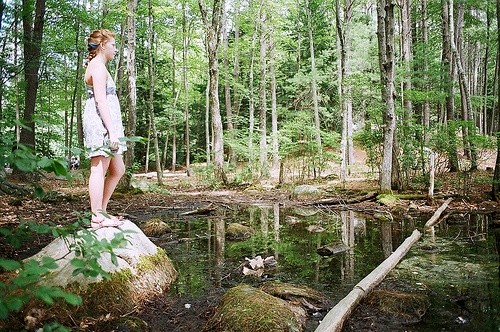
[90,214,123,231]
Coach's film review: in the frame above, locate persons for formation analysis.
[81,28,128,230]
